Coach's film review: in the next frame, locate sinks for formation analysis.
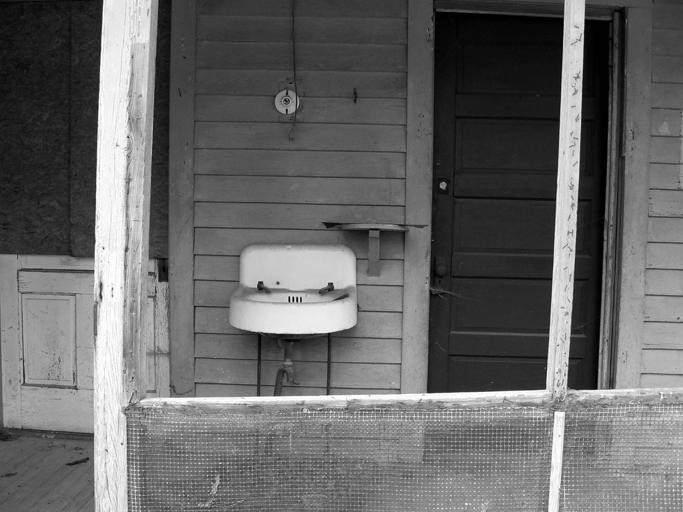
[227,242,358,337]
